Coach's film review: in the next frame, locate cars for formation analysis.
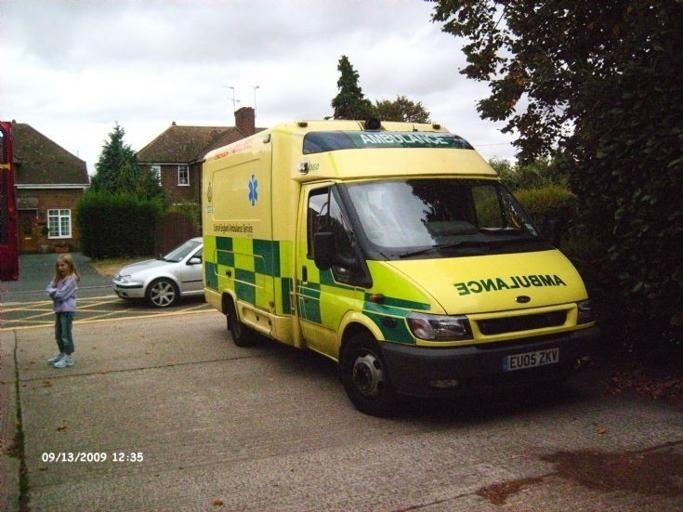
[111,236,205,309]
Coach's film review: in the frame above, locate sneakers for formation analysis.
[47,351,75,369]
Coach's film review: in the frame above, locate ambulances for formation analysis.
[199,116,600,418]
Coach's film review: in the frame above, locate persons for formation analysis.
[43,253,80,370]
